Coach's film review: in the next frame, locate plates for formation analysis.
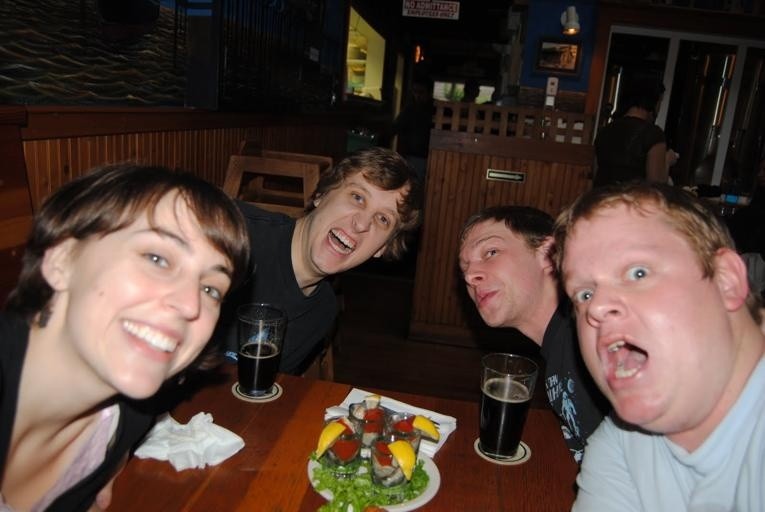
[308,449,441,511]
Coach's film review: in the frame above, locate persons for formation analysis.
[396,74,429,173]
[544,181,765,512]
[455,201,612,467]
[3,161,250,511]
[592,77,680,184]
[212,148,421,375]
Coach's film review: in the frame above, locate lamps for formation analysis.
[560,0,582,36]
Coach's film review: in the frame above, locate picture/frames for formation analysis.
[532,36,585,79]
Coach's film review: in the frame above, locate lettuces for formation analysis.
[312,454,428,512]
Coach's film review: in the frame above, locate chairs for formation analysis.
[222,151,333,382]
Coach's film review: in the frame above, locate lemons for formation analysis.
[315,421,346,460]
[387,439,417,481]
[413,413,440,442]
[365,394,384,404]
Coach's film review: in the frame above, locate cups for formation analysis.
[326,401,421,487]
[479,352,538,461]
[237,304,286,396]
